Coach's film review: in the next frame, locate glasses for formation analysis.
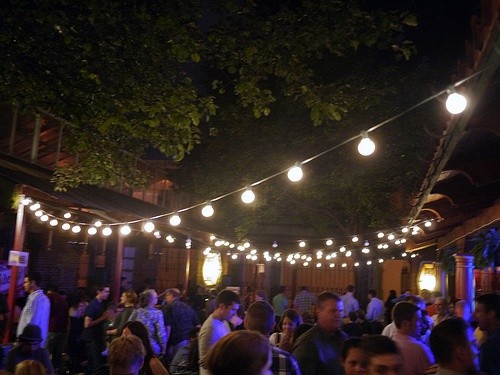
[105,291,110,292]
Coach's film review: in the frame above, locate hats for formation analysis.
[392,294,408,303]
[19,324,43,342]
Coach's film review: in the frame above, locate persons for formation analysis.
[46,273,500,375]
[0,370,12,375]
[12,270,52,349]
[13,359,48,375]
[0,293,23,342]
[7,324,56,375]
